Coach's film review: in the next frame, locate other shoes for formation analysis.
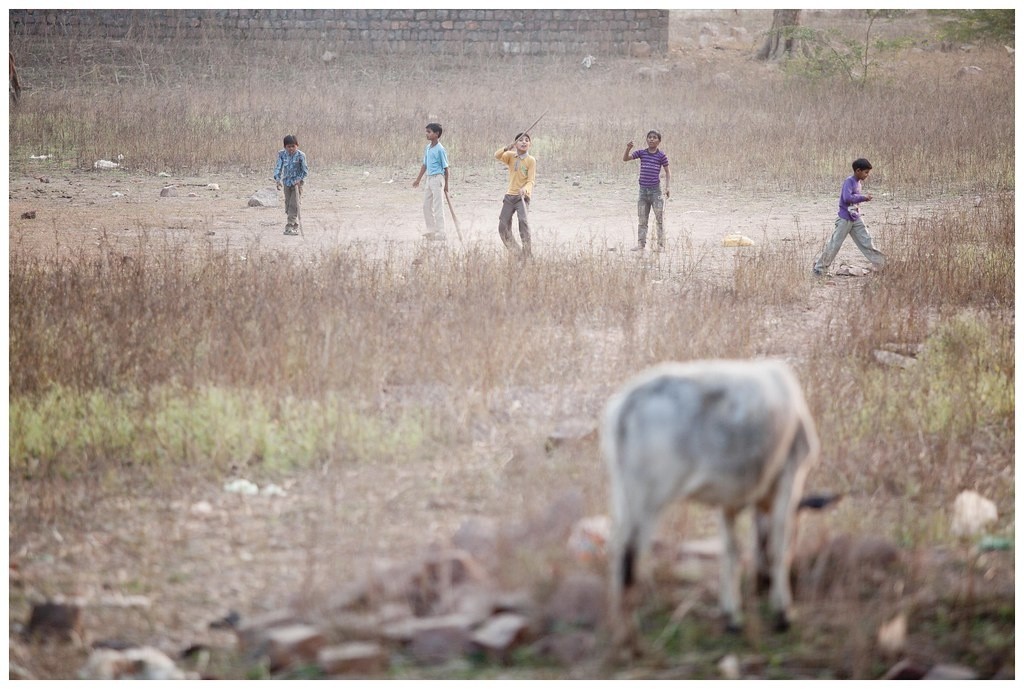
[424,231,446,240]
[816,270,832,277]
[631,246,644,250]
[653,244,665,252]
[283,224,298,235]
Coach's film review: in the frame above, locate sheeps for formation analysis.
[600,357,844,658]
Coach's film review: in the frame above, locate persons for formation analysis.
[813,158,887,275]
[623,129,671,253]
[412,122,449,239]
[274,135,308,235]
[494,133,536,256]
